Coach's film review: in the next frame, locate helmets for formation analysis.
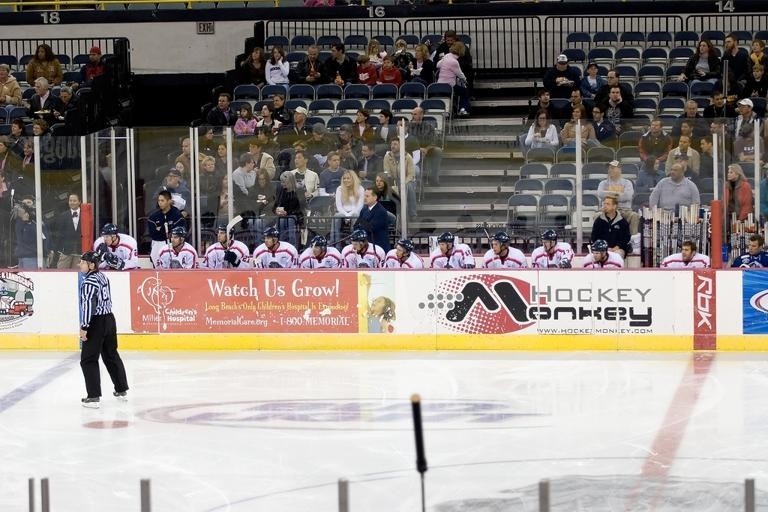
[101,224,119,235]
[80,251,99,264]
[310,236,327,247]
[398,240,413,253]
[541,229,557,241]
[437,231,454,243]
[172,226,187,238]
[590,239,608,251]
[264,228,280,239]
[491,231,510,245]
[349,230,368,241]
[219,223,235,233]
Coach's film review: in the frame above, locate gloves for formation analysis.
[224,251,241,266]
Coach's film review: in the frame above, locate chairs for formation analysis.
[506,32,767,246]
[0,51,127,248]
[149,32,472,242]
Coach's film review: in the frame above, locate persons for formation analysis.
[150,33,473,252]
[1,135,82,270]
[154,227,200,268]
[731,235,767,269]
[79,143,112,241]
[252,225,300,269]
[532,229,575,269]
[148,190,188,268]
[204,224,251,268]
[299,236,342,270]
[431,232,475,269]
[584,239,625,270]
[341,230,386,269]
[79,250,130,403]
[482,231,529,269]
[524,35,767,251]
[92,222,138,268]
[386,239,425,269]
[0,44,114,172]
[662,241,710,268]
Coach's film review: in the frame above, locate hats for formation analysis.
[90,47,100,54]
[557,54,567,64]
[605,160,623,168]
[292,106,305,115]
[737,98,753,108]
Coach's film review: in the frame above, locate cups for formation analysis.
[318,187,327,197]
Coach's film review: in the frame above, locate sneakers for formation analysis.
[113,390,126,396]
[82,396,99,403]
